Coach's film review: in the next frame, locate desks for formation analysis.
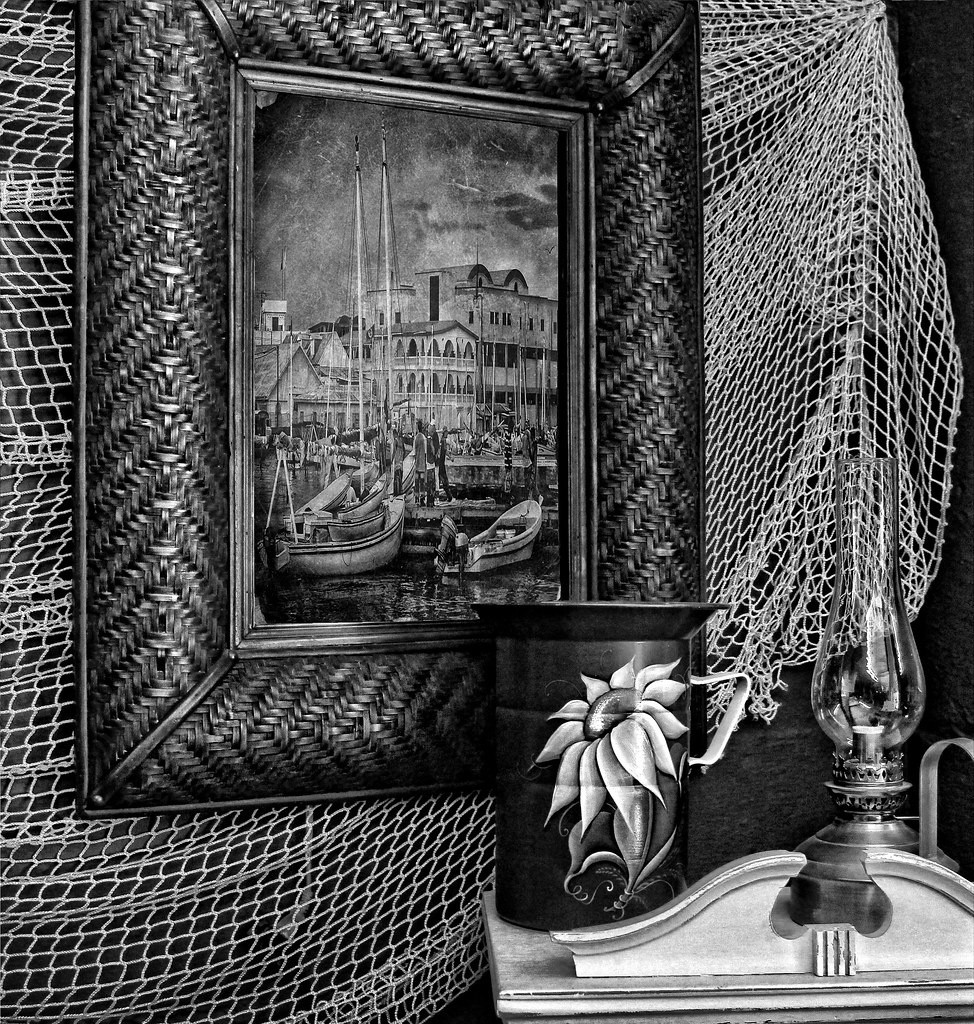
[479,891,974,1024]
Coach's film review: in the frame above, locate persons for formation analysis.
[387,422,544,509]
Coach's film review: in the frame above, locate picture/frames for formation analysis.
[72,0,709,823]
